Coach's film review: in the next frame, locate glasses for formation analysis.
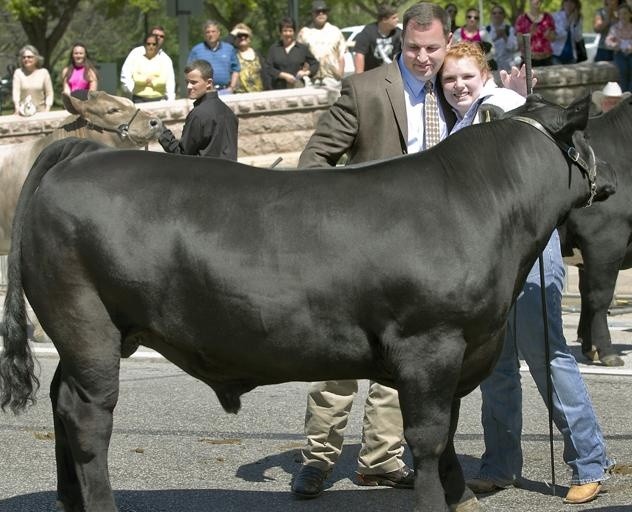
[468,16,478,19]
[147,43,156,45]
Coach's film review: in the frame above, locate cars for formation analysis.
[581,32,600,50]
[0,63,16,94]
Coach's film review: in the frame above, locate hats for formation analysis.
[314,1,327,11]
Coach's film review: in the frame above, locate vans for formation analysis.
[338,24,404,51]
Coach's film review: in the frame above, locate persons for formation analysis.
[120,25,176,104]
[158,60,238,162]
[13,44,54,115]
[187,0,402,95]
[62,43,97,97]
[445,0,632,93]
[439,42,616,504]
[291,2,538,499]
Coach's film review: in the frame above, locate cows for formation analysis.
[0,95,619,512]
[556,94,632,366]
[0,90,164,344]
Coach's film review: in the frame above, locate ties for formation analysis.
[424,81,440,149]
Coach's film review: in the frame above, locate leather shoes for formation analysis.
[466,478,496,492]
[355,465,414,488]
[564,482,602,503]
[292,465,324,498]
